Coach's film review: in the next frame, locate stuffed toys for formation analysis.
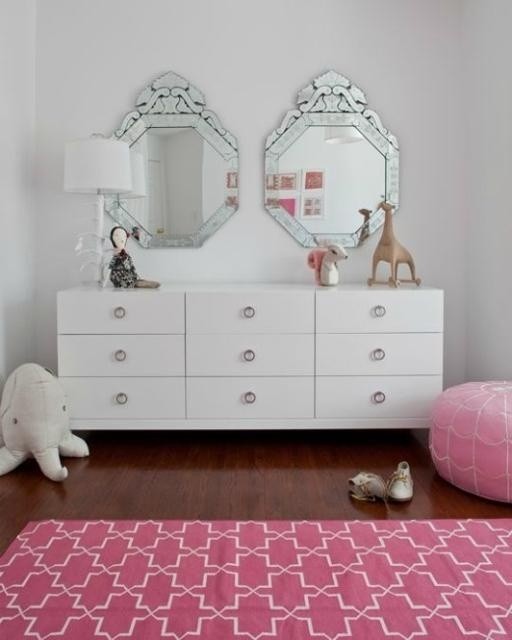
[108,226,161,290]
[0,363,90,482]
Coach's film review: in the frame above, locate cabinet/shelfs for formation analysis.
[54,282,444,431]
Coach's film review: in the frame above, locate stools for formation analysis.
[428,379,512,503]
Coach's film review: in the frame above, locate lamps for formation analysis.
[323,125,363,146]
[113,149,147,200]
[62,133,131,289]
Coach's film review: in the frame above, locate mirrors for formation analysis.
[264,70,400,249]
[104,70,240,250]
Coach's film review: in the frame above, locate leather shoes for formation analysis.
[347,462,414,501]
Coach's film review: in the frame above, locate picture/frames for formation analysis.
[278,172,297,192]
[277,196,297,218]
[300,193,325,219]
[223,170,239,190]
[301,168,325,192]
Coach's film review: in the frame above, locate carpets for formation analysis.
[0,517,512,640]
